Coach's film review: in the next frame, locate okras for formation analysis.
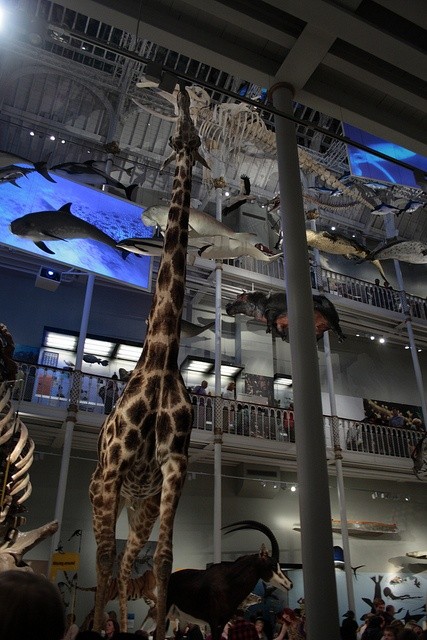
[10,203,130,261]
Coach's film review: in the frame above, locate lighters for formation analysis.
[89,83,210,639]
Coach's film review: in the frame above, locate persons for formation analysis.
[342,611,359,639]
[389,408,403,457]
[284,403,295,442]
[382,281,395,311]
[104,619,120,640]
[222,381,236,434]
[191,380,208,430]
[105,374,118,415]
[370,279,384,308]
[357,597,427,640]
[423,296,427,320]
[222,598,305,640]
[165,612,181,640]
[64,613,79,640]
[408,418,424,458]
